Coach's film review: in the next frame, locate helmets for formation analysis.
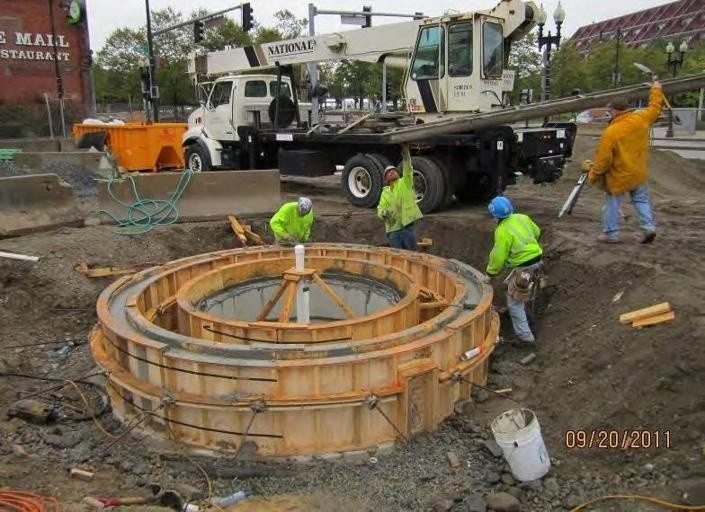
[384,166,400,176]
[488,196,513,218]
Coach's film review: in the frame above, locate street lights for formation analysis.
[665,40,688,138]
[535,0,566,123]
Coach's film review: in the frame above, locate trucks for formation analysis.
[176,0,580,219]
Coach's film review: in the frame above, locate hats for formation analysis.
[606,95,630,110]
[298,197,313,217]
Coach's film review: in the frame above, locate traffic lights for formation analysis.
[194,20,203,43]
[244,2,254,32]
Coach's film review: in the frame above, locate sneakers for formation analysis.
[638,229,657,245]
[511,339,535,348]
[597,234,622,242]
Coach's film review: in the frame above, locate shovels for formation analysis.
[633,63,683,125]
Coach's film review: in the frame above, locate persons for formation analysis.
[483,196,544,347]
[377,145,424,252]
[269,197,315,245]
[588,81,664,244]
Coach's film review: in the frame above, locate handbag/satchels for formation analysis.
[507,272,534,302]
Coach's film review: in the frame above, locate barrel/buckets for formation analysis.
[492,406,551,482]
